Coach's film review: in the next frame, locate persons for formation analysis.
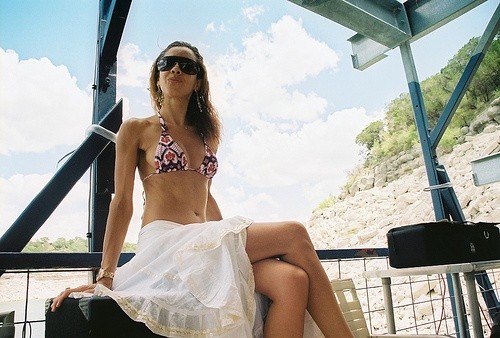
[51,42,356,337]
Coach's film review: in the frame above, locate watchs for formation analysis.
[96,269,115,281]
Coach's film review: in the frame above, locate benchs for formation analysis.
[43,291,272,337]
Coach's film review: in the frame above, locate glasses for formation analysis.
[156,55,201,75]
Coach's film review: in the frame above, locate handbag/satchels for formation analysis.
[386,217,500,269]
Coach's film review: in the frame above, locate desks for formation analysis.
[362,259,500,338]
